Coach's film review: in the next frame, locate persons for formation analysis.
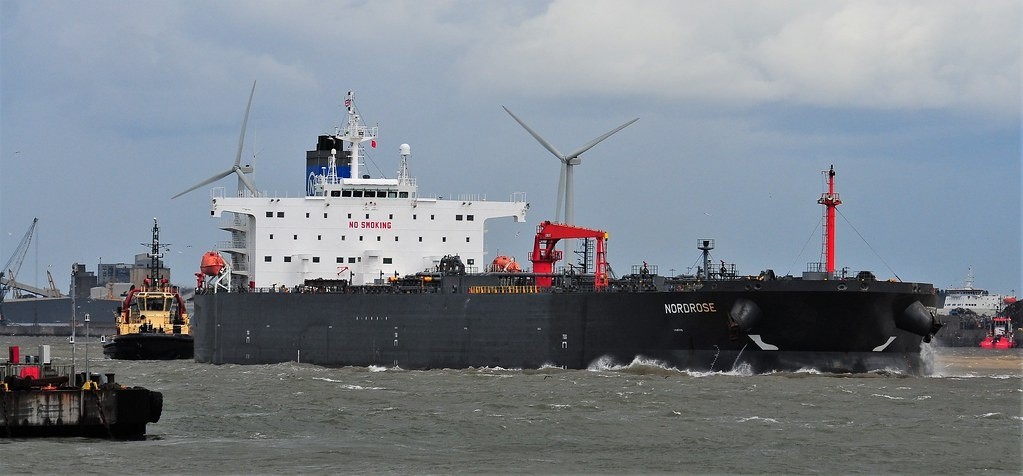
[121,383,130,389]
[43,383,58,390]
[81,379,98,390]
[0,380,9,391]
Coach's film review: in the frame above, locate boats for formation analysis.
[188,87,952,375]
[0,343,165,441]
[99,215,194,361]
[979,316,1017,349]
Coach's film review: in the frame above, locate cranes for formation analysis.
[0,215,38,299]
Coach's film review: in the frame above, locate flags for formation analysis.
[372,141,377,148]
[345,100,350,106]
